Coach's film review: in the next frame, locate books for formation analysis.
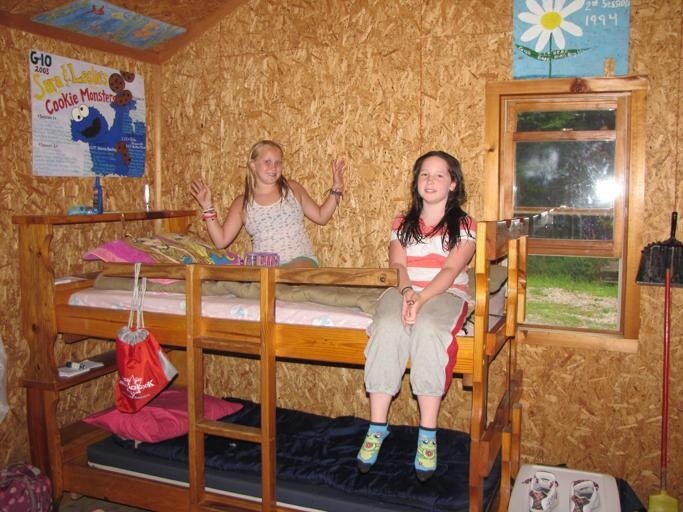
[55,359,104,377]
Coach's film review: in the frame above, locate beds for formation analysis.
[12,209,529,512]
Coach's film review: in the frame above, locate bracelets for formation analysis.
[328,184,344,197]
[201,206,218,221]
[401,286,413,295]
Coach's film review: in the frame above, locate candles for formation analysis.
[145,185,149,202]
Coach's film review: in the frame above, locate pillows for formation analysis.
[82,388,244,443]
[82,233,244,285]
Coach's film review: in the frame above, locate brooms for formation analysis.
[648,266,679,512]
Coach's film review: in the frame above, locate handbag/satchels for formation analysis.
[0,461,53,512]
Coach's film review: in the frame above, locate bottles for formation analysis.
[143,183,152,211]
[92,176,103,213]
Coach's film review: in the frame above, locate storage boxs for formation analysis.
[507,463,621,512]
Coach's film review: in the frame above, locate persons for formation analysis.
[187,140,348,270]
[355,151,478,482]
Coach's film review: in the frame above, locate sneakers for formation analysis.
[522,472,561,512]
[568,479,601,512]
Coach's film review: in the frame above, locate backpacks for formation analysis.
[113,323,178,414]
